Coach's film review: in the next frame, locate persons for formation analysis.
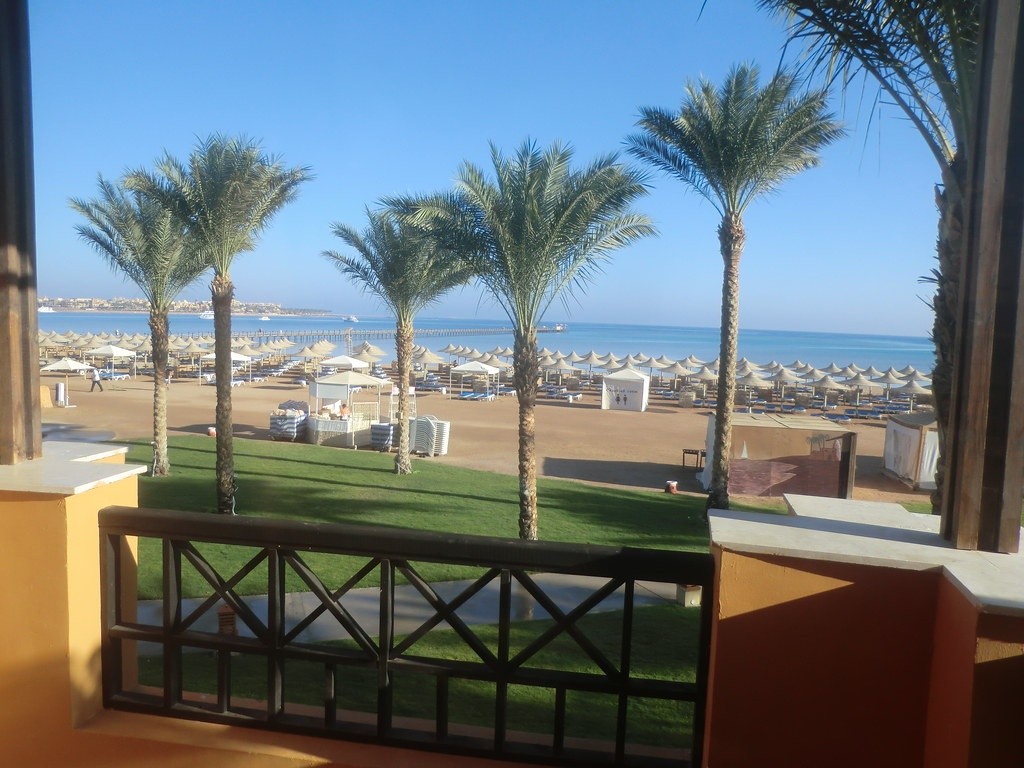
[90,368,103,393]
[341,403,349,416]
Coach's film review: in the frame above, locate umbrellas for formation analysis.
[38,328,934,412]
[41,356,94,407]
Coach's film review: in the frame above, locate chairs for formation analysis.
[655,386,916,423]
[97,358,300,387]
[322,364,593,402]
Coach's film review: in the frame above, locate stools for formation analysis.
[682,449,699,468]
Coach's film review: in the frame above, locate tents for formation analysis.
[85,344,136,380]
[318,355,368,377]
[601,369,650,412]
[882,412,940,484]
[449,361,500,401]
[199,351,252,386]
[700,412,857,499]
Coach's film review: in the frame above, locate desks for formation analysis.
[700,449,706,469]
